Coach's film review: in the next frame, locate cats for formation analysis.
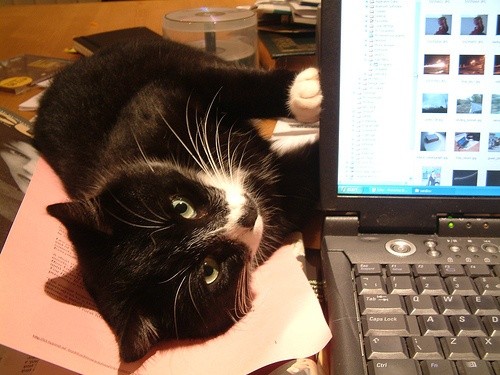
[33,39,324,363]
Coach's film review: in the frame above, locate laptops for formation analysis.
[317,0,500,375]
